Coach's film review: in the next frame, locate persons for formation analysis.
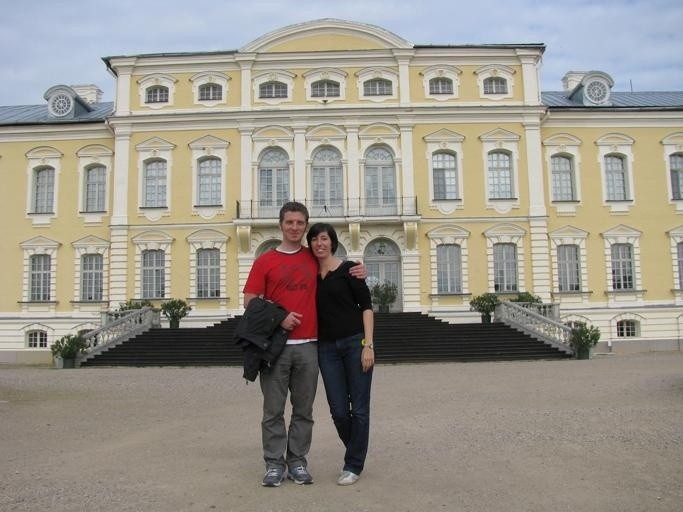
[242,201,368,487]
[265,222,375,486]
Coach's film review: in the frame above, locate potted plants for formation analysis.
[161,300,192,328]
[469,294,502,324]
[51,335,87,368]
[568,321,600,359]
[370,280,398,314]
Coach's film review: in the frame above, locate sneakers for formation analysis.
[337,470,360,485]
[260,467,288,486]
[287,465,313,486]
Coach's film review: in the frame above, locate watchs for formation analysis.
[364,343,374,350]
